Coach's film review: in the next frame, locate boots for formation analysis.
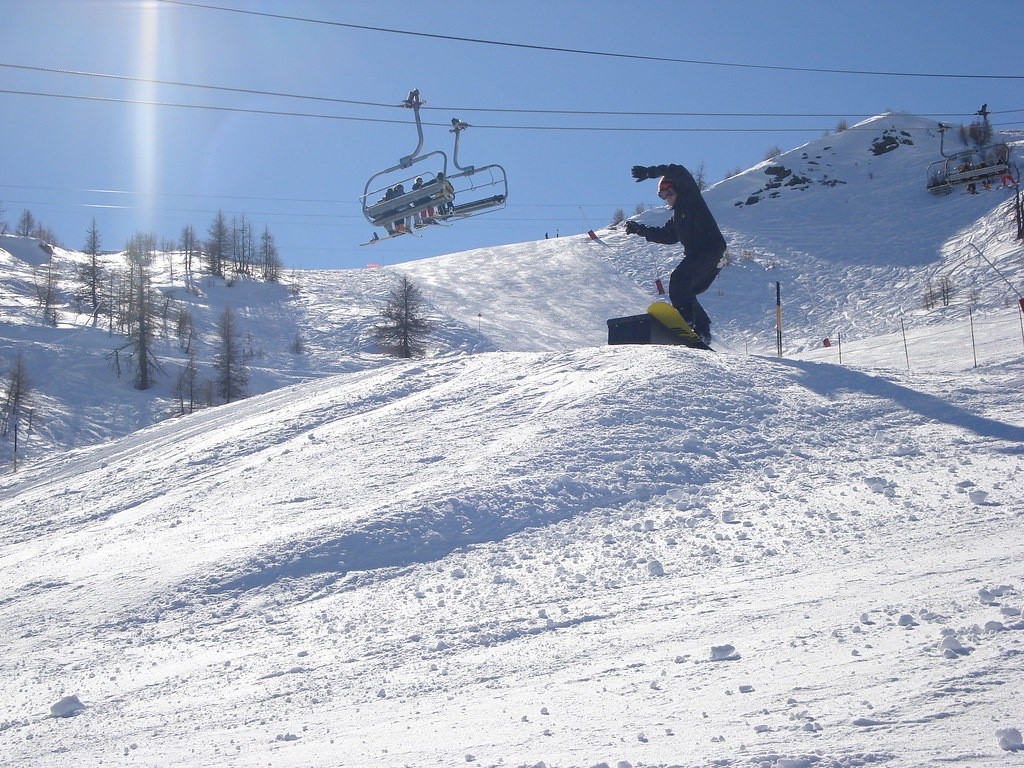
[396,224,404,231]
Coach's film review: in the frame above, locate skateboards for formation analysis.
[647,301,719,354]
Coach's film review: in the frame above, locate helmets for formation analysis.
[658,175,675,199]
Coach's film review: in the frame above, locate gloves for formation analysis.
[624,221,639,235]
[631,166,648,182]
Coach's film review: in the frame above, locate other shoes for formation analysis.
[698,334,712,345]
[450,211,454,215]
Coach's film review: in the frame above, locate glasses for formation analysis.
[657,185,675,200]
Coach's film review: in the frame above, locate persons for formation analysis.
[959,156,1016,195]
[623,164,728,344]
[376,172,455,234]
